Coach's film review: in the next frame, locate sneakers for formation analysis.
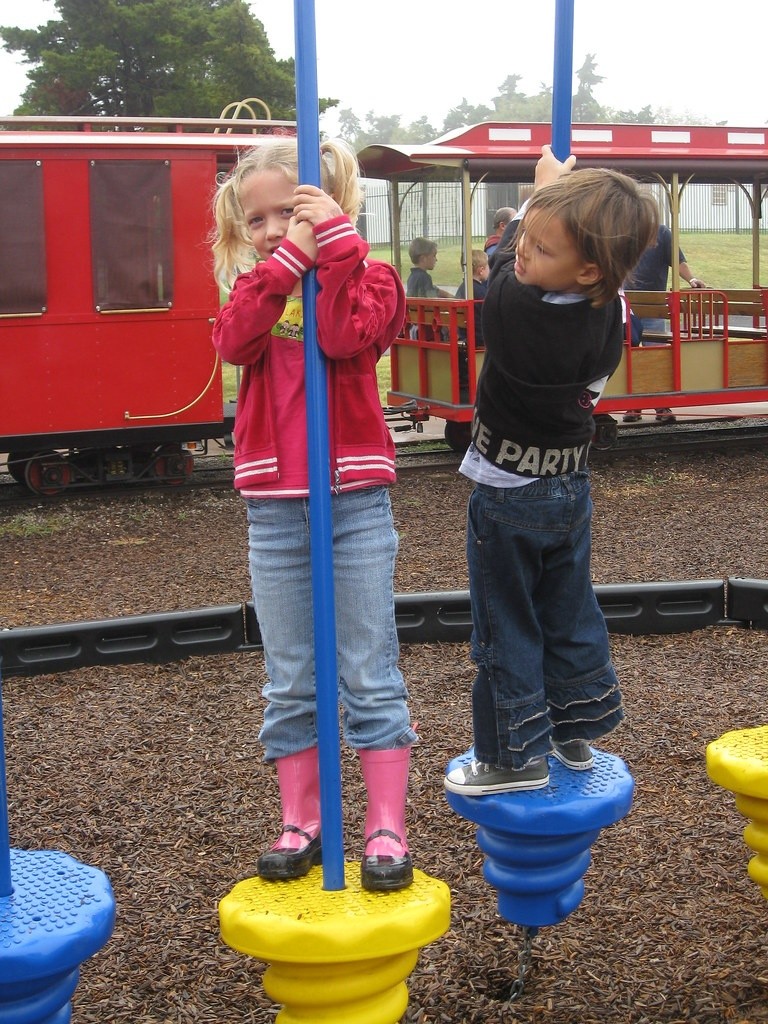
[444,756,549,796]
[623,410,642,421]
[655,408,676,422]
[552,741,596,770]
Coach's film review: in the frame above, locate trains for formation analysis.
[2,113,768,500]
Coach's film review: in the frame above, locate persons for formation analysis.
[484,206,516,255]
[405,238,454,298]
[212,137,415,891]
[444,144,657,795]
[451,251,490,346]
[623,225,707,423]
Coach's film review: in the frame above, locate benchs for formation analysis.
[670,287,768,342]
[399,297,486,351]
[623,290,724,344]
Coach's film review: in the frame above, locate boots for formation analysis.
[356,746,413,888]
[256,745,322,878]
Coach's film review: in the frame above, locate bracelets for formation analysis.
[689,278,696,283]
